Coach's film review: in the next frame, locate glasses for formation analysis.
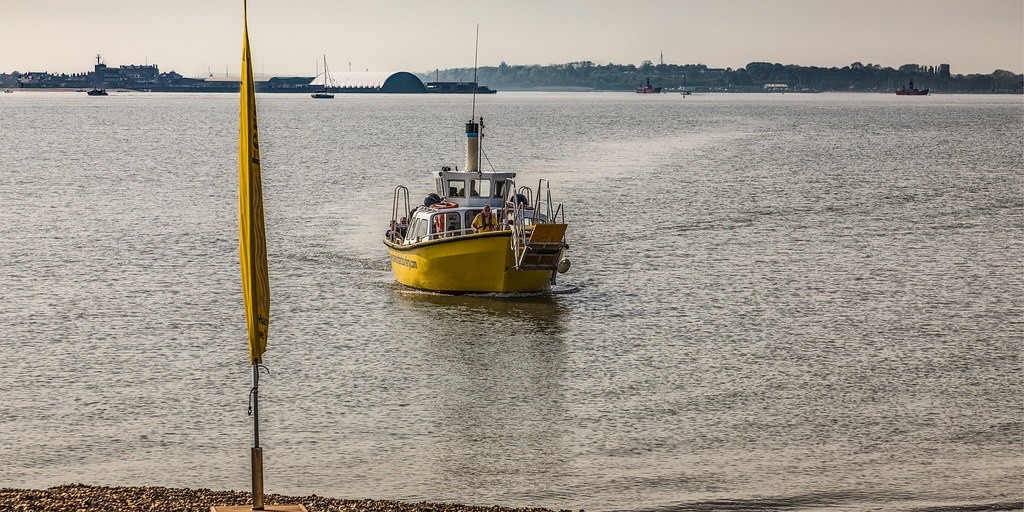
[484,210,490,212]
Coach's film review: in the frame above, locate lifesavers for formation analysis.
[429,202,459,209]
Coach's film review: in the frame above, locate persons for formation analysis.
[470,204,499,233]
[384,216,408,242]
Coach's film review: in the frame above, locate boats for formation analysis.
[383,29,571,293]
[636,78,662,93]
[87,87,109,95]
[76,89,131,92]
[424,68,497,93]
[895,77,929,95]
[4,88,13,93]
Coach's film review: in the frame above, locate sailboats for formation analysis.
[311,55,334,98]
